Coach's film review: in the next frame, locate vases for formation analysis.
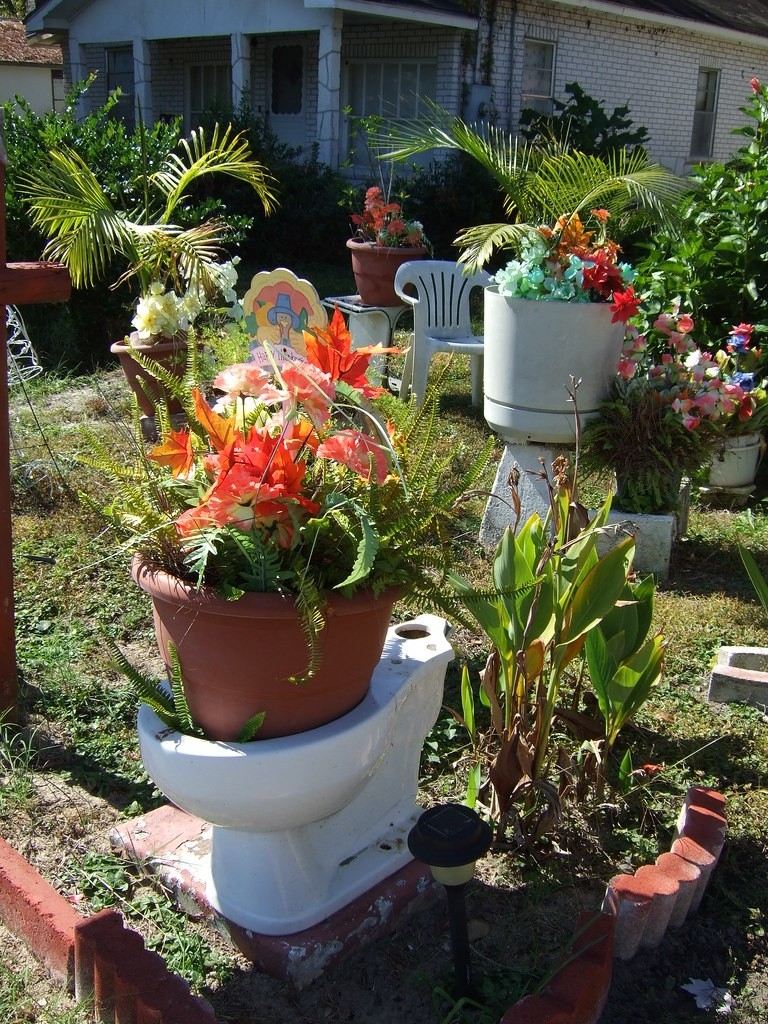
[130,543,413,742]
[578,474,696,580]
[482,285,627,444]
[346,237,430,308]
[110,339,191,418]
[697,432,762,489]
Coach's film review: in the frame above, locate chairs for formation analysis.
[394,259,493,411]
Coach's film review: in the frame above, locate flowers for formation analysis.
[15,94,282,345]
[78,254,499,686]
[570,288,768,513]
[485,208,640,302]
[351,186,426,248]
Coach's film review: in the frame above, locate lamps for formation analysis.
[407,802,493,887]
